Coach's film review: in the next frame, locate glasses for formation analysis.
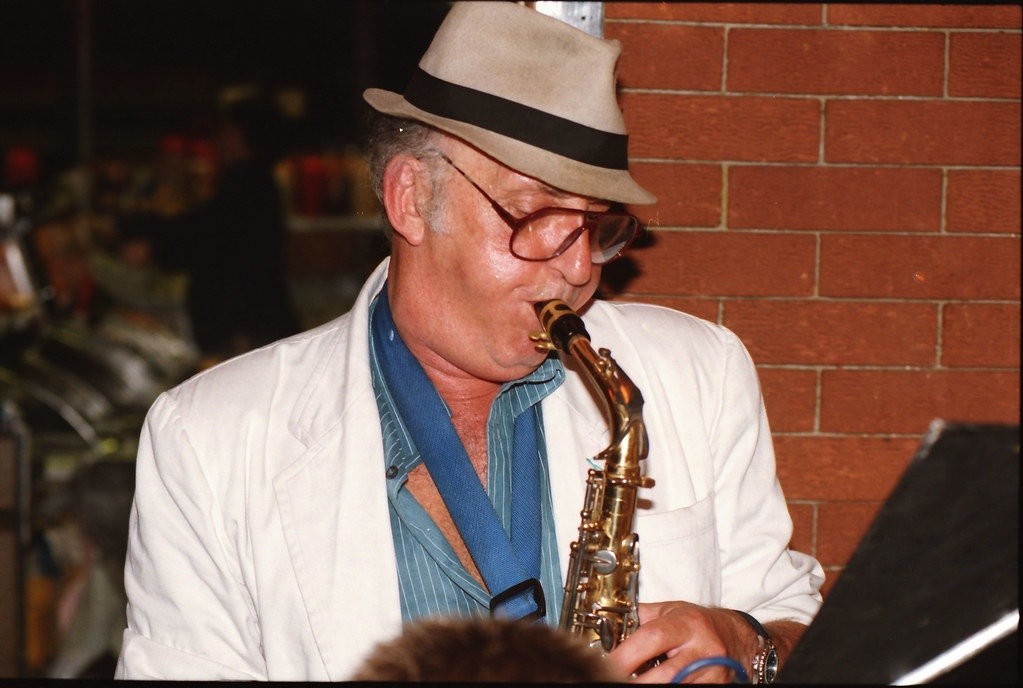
[442,152,640,264]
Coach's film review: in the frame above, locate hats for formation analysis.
[363,0,657,202]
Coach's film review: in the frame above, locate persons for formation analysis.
[341,622,623,688]
[116,1,824,687]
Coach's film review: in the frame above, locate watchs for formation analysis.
[732,610,779,686]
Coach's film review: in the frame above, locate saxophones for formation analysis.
[526,294,655,660]
